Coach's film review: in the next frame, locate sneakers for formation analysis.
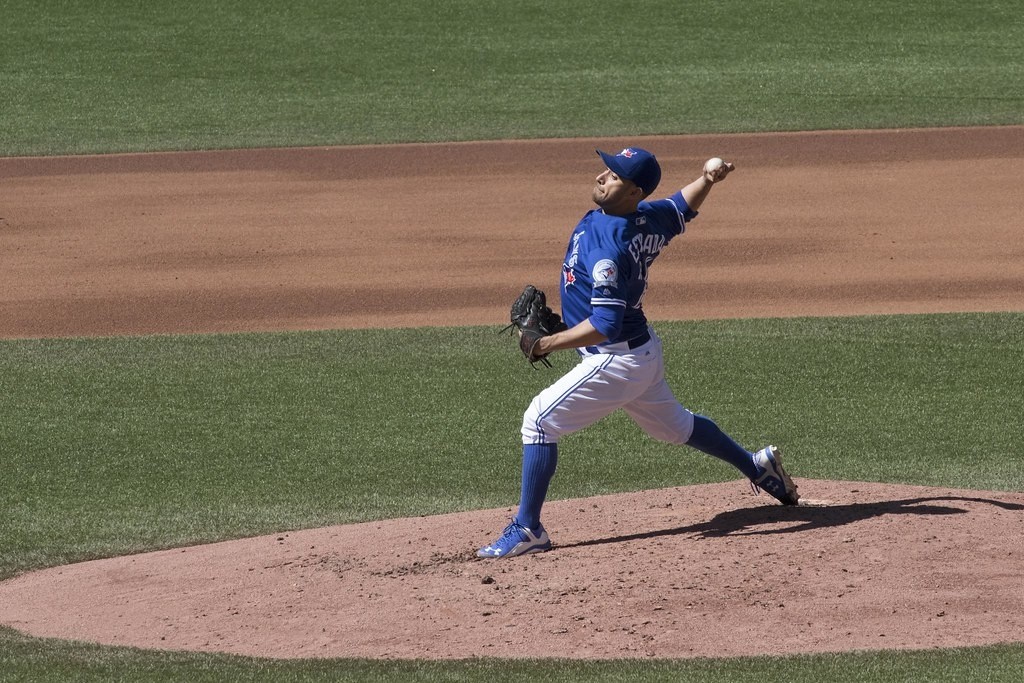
[750,444,800,506]
[476,515,552,557]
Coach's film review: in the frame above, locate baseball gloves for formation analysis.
[510,283,568,362]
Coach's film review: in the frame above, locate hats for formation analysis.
[595,148,661,195]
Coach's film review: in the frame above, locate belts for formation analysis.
[576,331,650,356]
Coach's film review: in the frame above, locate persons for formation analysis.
[476,147,801,561]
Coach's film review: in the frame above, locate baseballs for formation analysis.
[706,157,725,176]
